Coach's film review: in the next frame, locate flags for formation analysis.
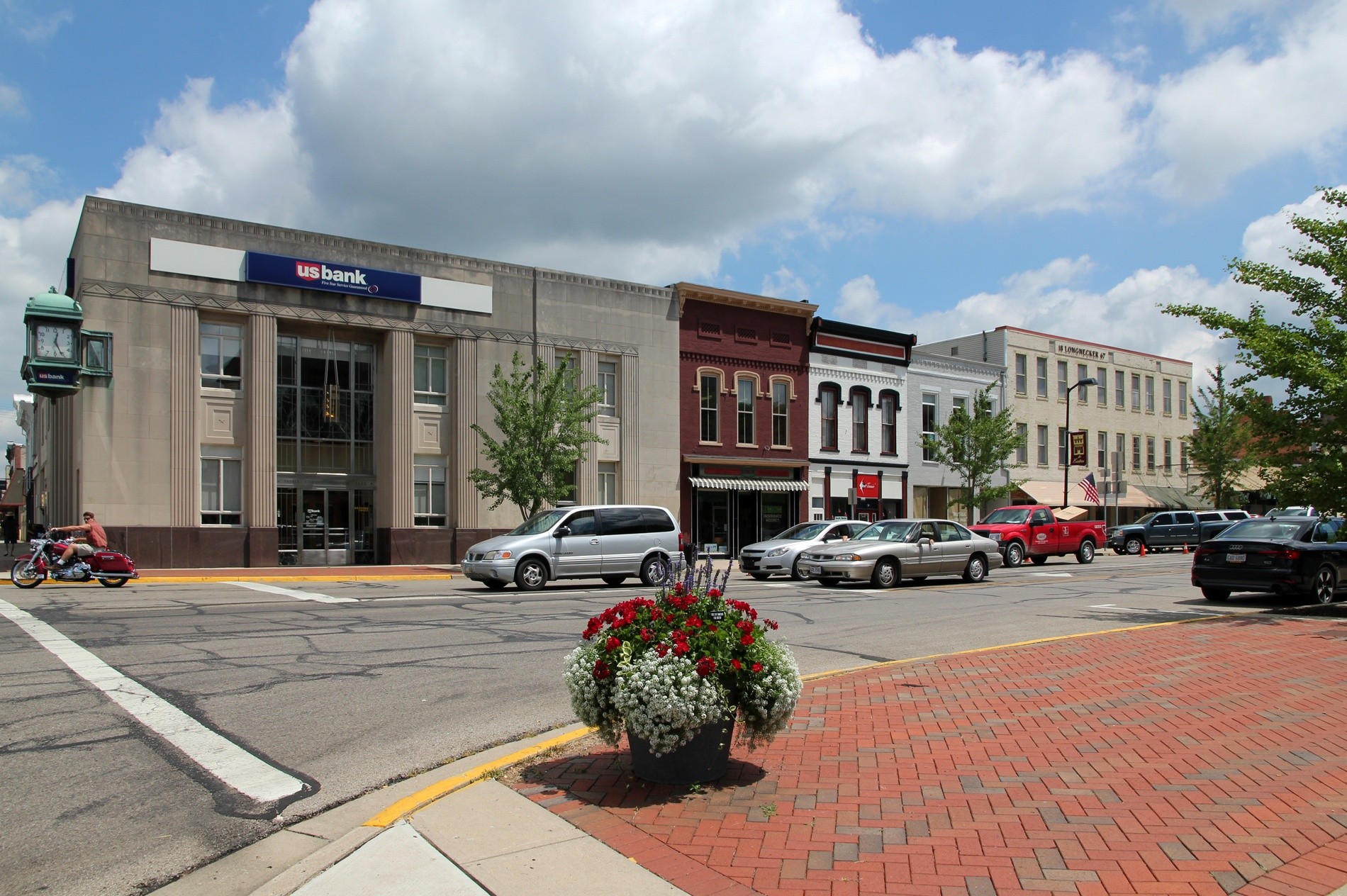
[1077,472,1100,507]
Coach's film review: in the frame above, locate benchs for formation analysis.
[940,533,958,541]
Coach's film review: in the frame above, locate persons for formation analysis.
[1,512,18,557]
[902,525,934,545]
[44,512,108,571]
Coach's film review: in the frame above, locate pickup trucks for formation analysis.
[964,505,1107,568]
[1111,510,1237,554]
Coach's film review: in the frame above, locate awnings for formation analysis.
[687,476,810,491]
[1010,479,1160,508]
[1135,485,1242,510]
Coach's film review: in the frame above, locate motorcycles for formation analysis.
[10,522,139,590]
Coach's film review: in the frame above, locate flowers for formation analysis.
[562,543,802,756]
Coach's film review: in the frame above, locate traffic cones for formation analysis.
[1140,544,1147,557]
[1182,543,1189,555]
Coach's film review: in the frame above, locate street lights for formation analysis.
[1064,378,1099,508]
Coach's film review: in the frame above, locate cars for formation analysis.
[1191,516,1347,604]
[1105,511,1158,547]
[740,519,1005,590]
[1264,506,1320,535]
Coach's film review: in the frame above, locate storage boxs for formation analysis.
[1040,507,1061,521]
[1055,505,1088,521]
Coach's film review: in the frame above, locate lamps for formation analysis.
[323,326,340,424]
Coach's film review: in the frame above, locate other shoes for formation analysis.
[4,553,8,556]
[43,565,62,572]
[10,554,14,556]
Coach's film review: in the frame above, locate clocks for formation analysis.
[37,327,73,359]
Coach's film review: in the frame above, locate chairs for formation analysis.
[838,525,849,537]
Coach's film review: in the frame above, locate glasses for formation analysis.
[84,517,91,520]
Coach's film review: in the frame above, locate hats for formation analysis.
[6,512,15,516]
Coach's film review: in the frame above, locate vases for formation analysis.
[626,707,739,784]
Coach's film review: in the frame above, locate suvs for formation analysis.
[1197,511,1252,522]
[460,505,683,590]
